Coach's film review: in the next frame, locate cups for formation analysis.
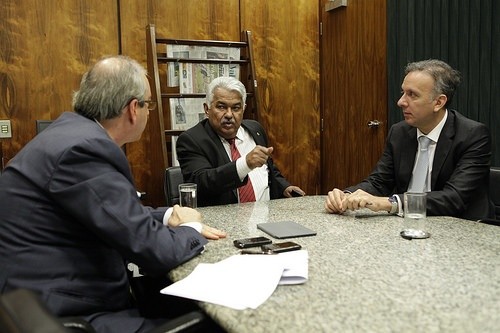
[403,191,427,238]
[177,183,198,212]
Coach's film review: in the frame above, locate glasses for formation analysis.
[144,100,157,110]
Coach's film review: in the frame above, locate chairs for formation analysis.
[0,286,202,333]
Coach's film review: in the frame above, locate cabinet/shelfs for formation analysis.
[145,23,263,206]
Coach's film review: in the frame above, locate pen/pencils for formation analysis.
[239,250,272,255]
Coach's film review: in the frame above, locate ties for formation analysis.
[407,137,432,193]
[228,138,257,203]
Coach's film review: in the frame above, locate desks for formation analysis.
[166,195,500,333]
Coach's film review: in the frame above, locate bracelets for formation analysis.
[386,197,398,213]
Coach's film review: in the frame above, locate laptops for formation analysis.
[259,222,317,239]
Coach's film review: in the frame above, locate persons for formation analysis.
[325,60,492,222]
[176,77,306,207]
[0,55,226,333]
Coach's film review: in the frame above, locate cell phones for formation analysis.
[261,241,301,253]
[234,236,272,248]
